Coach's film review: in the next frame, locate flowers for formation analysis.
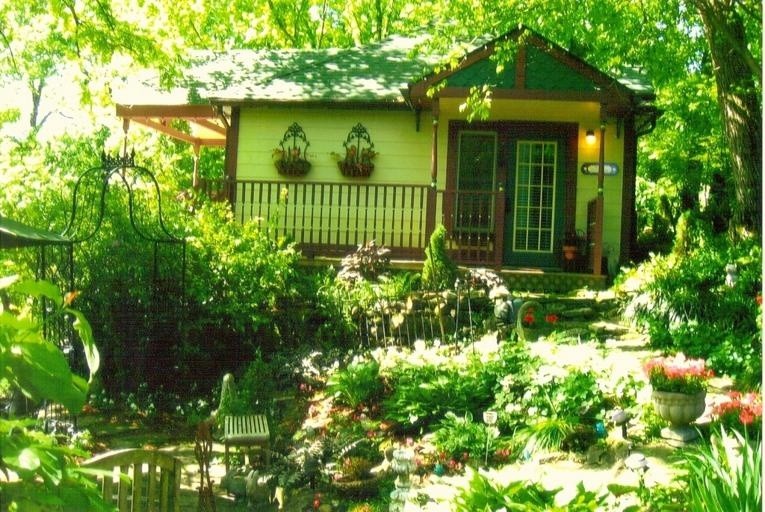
[343,145,373,167]
[643,350,709,394]
[272,148,303,164]
[710,392,763,426]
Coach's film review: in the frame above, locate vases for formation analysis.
[654,394,706,441]
[710,432,762,471]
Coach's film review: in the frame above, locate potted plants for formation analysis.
[556,230,587,262]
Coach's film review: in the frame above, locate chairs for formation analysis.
[61,449,179,511]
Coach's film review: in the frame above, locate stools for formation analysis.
[223,411,272,476]
